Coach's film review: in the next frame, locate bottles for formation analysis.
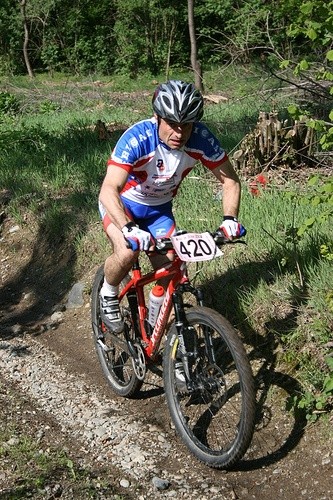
[149,284,165,326]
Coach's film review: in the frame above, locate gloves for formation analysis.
[120,221,156,252]
[214,216,246,241]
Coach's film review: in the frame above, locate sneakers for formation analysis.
[97,288,124,334]
[173,358,187,383]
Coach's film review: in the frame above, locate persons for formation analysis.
[98,80,241,381]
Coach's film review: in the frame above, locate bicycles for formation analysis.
[92,225,255,470]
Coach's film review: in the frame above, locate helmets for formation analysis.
[151,79,204,124]
